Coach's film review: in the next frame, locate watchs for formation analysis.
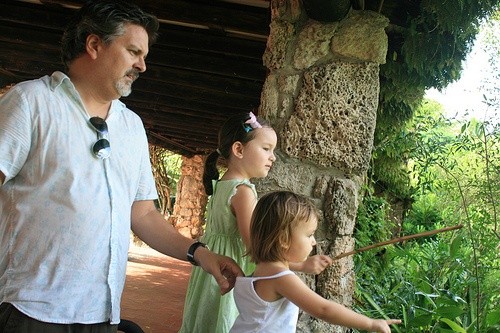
[187,242,211,267]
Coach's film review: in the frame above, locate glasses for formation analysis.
[87,116,112,158]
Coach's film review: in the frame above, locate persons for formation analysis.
[179,112,278,333]
[0,0,245,333]
[230,190,401,333]
